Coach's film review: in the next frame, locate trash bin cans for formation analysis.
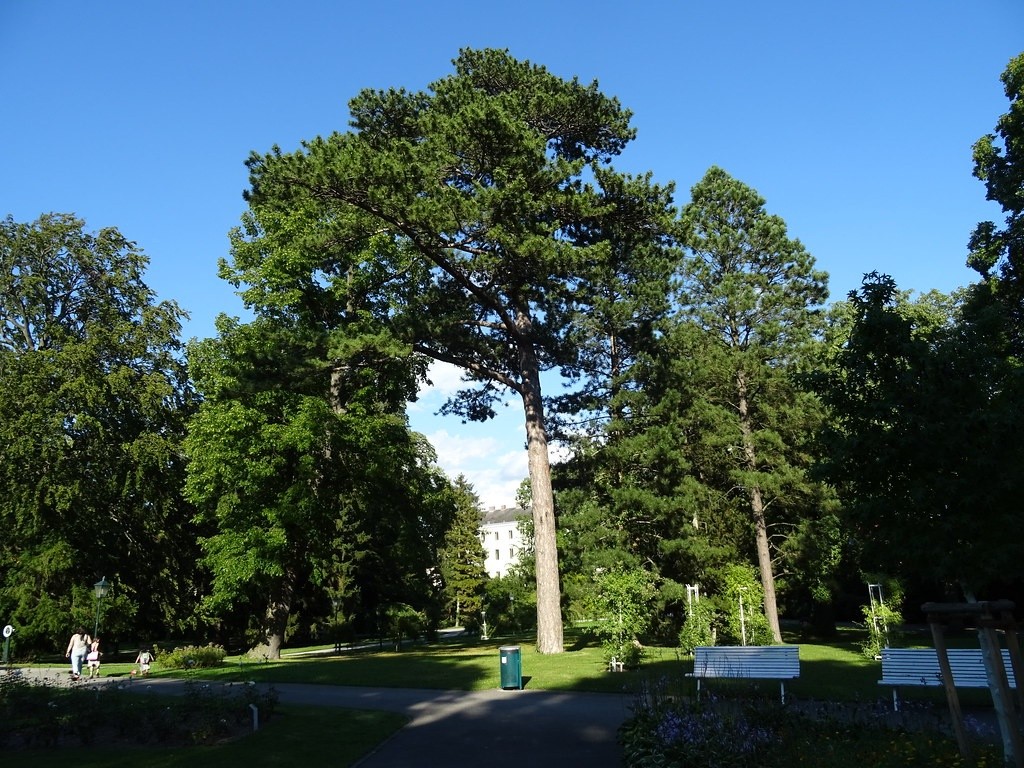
[500,646,522,688]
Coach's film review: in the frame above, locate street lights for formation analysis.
[93,575,112,641]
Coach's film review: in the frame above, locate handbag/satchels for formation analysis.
[86,650,100,661]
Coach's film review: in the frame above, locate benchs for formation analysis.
[684,643,803,707]
[876,646,1016,720]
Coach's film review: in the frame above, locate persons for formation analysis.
[88,636,103,679]
[135,649,154,676]
[66,625,92,680]
